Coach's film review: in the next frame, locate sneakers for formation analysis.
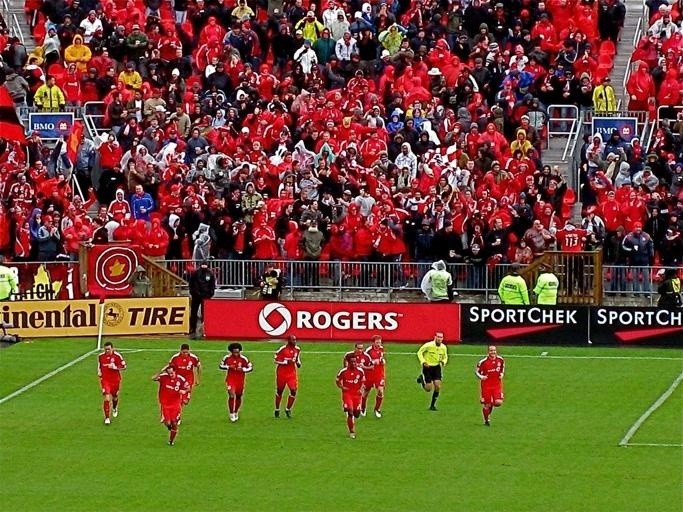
[484,420,490,427]
[349,431,356,439]
[104,415,110,424]
[429,406,437,411]
[229,412,239,422]
[113,407,118,417]
[416,374,423,384]
[168,440,174,446]
[274,408,280,419]
[374,408,381,418]
[285,407,292,419]
[360,409,367,417]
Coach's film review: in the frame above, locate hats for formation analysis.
[510,263,520,270]
[268,263,276,268]
[540,262,553,270]
[0,0,678,246]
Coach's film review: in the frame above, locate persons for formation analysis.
[414,330,448,413]
[158,342,202,407]
[341,341,374,385]
[361,333,386,420]
[94,338,126,426]
[149,364,192,449]
[472,344,506,428]
[333,356,366,441]
[2,0,683,308]
[270,334,304,415]
[186,260,216,335]
[216,341,253,423]
